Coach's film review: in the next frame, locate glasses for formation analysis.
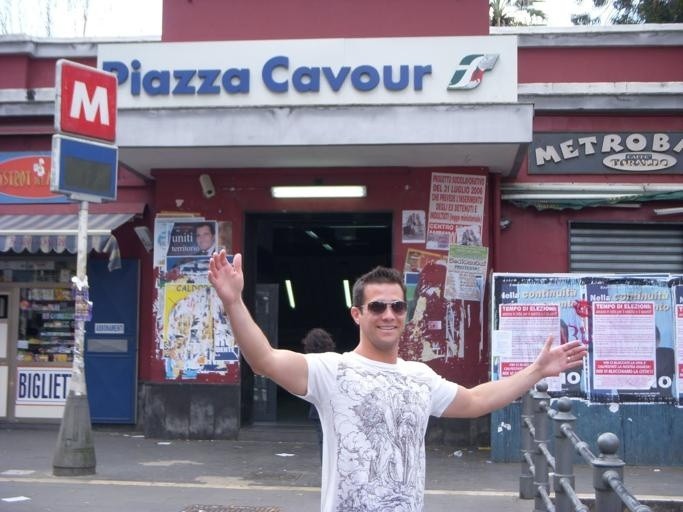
[358,301,408,314]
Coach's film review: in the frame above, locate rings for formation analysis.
[567,357,571,361]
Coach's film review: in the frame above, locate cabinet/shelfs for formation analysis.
[17,286,77,365]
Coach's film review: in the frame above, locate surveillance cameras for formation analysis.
[198,174,215,198]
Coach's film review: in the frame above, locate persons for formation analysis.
[194,223,215,255]
[208,249,587,512]
